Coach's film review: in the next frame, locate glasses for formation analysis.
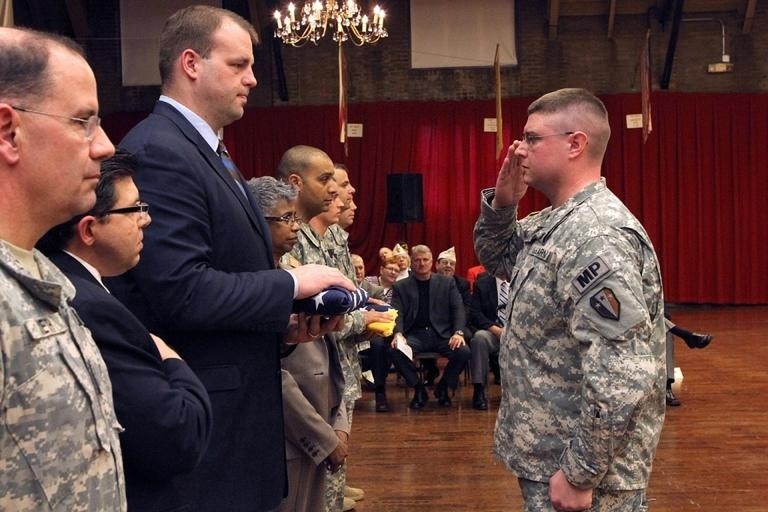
[266,215,303,224]
[523,131,588,146]
[13,104,101,141]
[105,202,148,217]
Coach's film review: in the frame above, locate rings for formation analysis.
[307,331,320,339]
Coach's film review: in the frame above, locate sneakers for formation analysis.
[342,486,364,511]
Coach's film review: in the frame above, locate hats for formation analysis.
[438,246,457,262]
[392,244,406,256]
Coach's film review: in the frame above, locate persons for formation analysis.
[237,175,349,510]
[49,146,210,512]
[117,4,358,512]
[362,241,491,384]
[663,316,714,407]
[347,255,392,409]
[277,143,391,511]
[390,245,467,408]
[0,25,130,512]
[469,274,525,411]
[473,85,666,512]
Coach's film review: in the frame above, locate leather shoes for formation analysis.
[687,332,712,349]
[375,366,499,412]
[666,390,681,406]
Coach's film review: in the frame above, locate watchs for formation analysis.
[455,329,464,336]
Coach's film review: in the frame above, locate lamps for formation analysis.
[272,0,388,47]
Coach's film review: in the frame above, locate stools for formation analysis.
[404,350,463,412]
[484,349,500,408]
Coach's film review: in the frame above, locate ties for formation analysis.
[216,140,241,183]
